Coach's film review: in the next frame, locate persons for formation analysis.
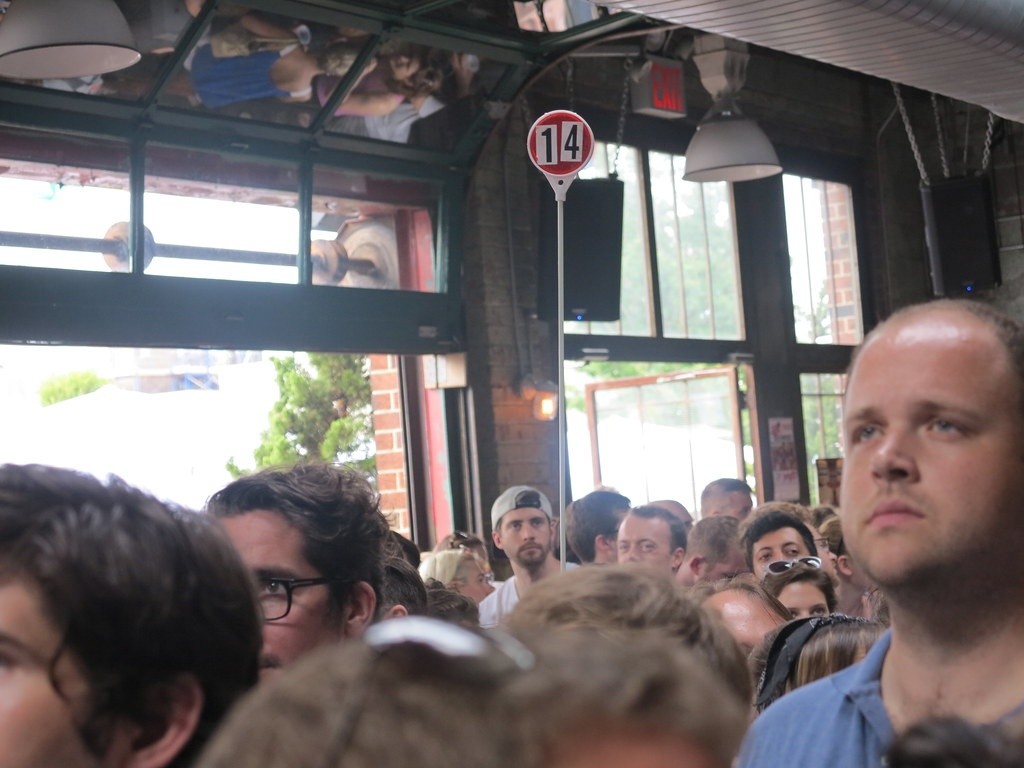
[1,463,202,768]
[738,298,1024,768]
[166,11,481,142]
[166,458,890,768]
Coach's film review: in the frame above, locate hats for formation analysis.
[492,484,553,558]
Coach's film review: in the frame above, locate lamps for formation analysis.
[680,34,782,183]
[0,0,140,79]
[518,375,556,421]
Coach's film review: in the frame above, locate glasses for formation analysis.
[760,556,824,587]
[248,571,338,620]
[316,615,537,768]
[457,544,469,580]
[450,530,469,550]
[814,537,830,553]
